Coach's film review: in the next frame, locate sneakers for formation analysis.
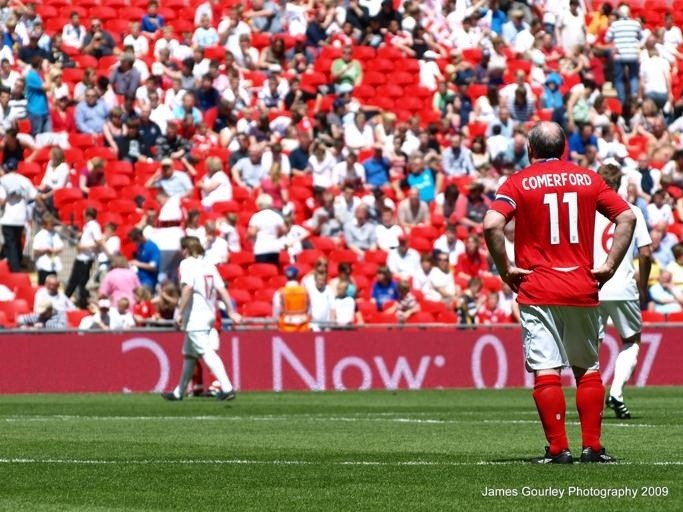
[605,396,631,419]
[530,446,573,464]
[216,390,235,400]
[581,447,617,462]
[160,392,182,400]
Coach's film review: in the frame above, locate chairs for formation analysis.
[0,0,682,331]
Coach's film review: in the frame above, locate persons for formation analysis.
[160,234,242,404]
[0,0,683,331]
[595,162,655,423]
[176,267,222,395]
[481,119,636,463]
